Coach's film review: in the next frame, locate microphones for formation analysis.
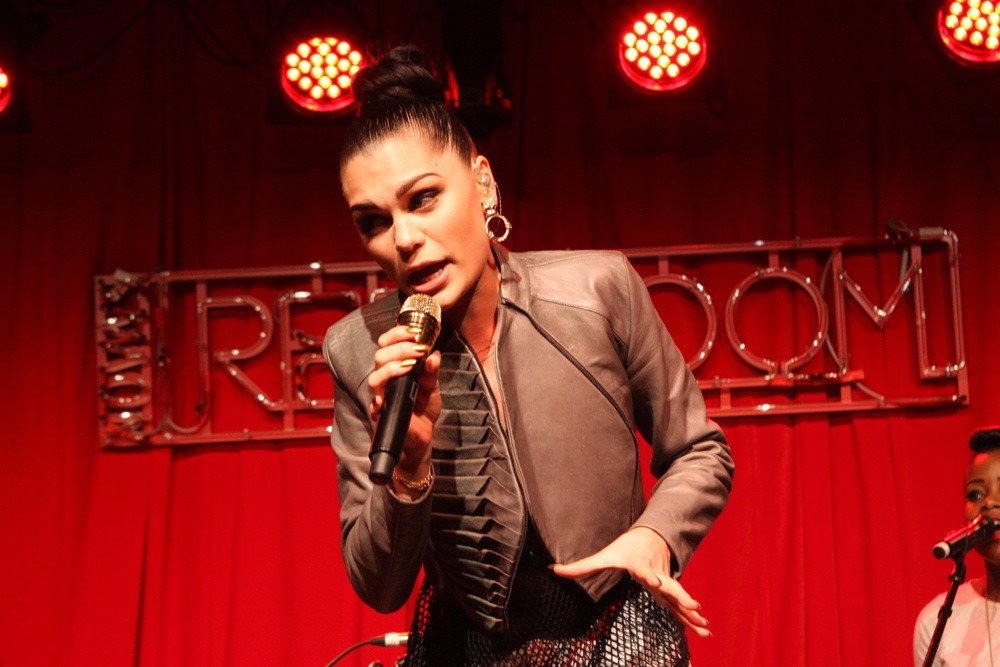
[930,512,995,561]
[365,292,442,486]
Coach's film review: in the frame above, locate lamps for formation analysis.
[265,0,391,125]
[616,0,718,104]
[929,0,1000,69]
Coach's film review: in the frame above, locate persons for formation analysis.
[913,428,1000,667]
[321,47,735,667]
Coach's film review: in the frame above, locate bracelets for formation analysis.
[391,465,433,492]
